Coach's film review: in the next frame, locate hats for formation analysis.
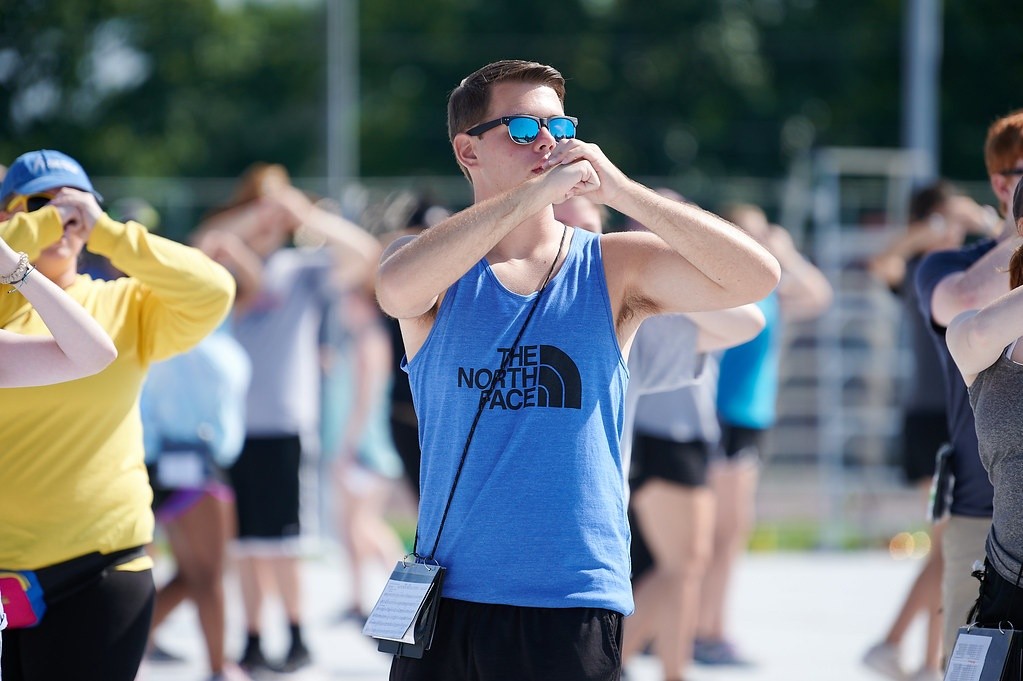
[1,149,103,205]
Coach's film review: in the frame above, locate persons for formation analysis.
[82,160,448,681]
[373,60,782,681]
[0,235,117,388]
[0,146,237,681]
[866,110,1023,681]
[550,187,831,681]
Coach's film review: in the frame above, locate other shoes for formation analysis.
[243,651,276,679]
[865,649,905,679]
[692,636,742,667]
[279,647,308,673]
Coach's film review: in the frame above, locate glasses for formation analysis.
[467,115,579,145]
[5,192,54,214]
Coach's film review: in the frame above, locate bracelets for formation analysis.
[0,251,37,294]
